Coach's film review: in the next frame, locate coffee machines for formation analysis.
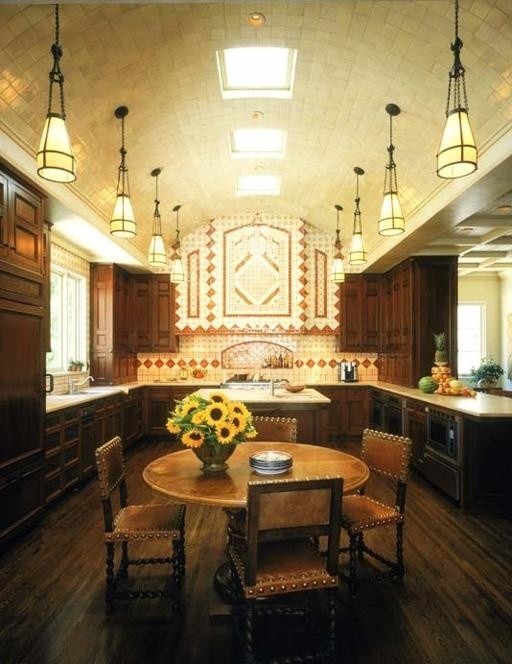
[340,361,358,382]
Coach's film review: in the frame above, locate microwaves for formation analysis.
[423,408,460,466]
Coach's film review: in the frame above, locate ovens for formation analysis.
[367,388,404,439]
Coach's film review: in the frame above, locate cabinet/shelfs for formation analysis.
[144,386,195,437]
[89,262,176,385]
[340,256,458,388]
[45,386,144,510]
[316,385,512,511]
[0,155,53,561]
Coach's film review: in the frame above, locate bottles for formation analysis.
[270,351,291,369]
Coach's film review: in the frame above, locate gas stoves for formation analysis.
[224,377,287,386]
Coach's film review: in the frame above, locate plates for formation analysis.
[249,450,294,475]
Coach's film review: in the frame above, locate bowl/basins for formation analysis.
[284,383,304,392]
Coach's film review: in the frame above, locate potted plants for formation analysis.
[69,361,83,372]
[470,353,504,389]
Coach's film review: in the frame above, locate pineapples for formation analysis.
[433,333,448,363]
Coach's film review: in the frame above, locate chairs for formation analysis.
[224,474,344,663]
[247,415,298,443]
[93,437,186,616]
[315,429,412,599]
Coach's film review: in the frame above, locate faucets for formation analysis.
[270,380,285,396]
[71,376,107,395]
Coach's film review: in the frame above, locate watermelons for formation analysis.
[418,377,438,392]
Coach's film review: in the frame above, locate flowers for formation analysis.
[165,392,260,449]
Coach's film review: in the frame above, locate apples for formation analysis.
[431,367,476,397]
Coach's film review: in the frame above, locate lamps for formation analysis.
[377,103,406,236]
[109,105,138,237]
[436,0,478,178]
[348,167,368,266]
[329,205,346,283]
[36,1,76,183]
[147,169,167,265]
[169,205,186,283]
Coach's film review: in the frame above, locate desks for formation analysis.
[142,441,371,606]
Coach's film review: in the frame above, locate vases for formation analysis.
[190,443,237,472]
